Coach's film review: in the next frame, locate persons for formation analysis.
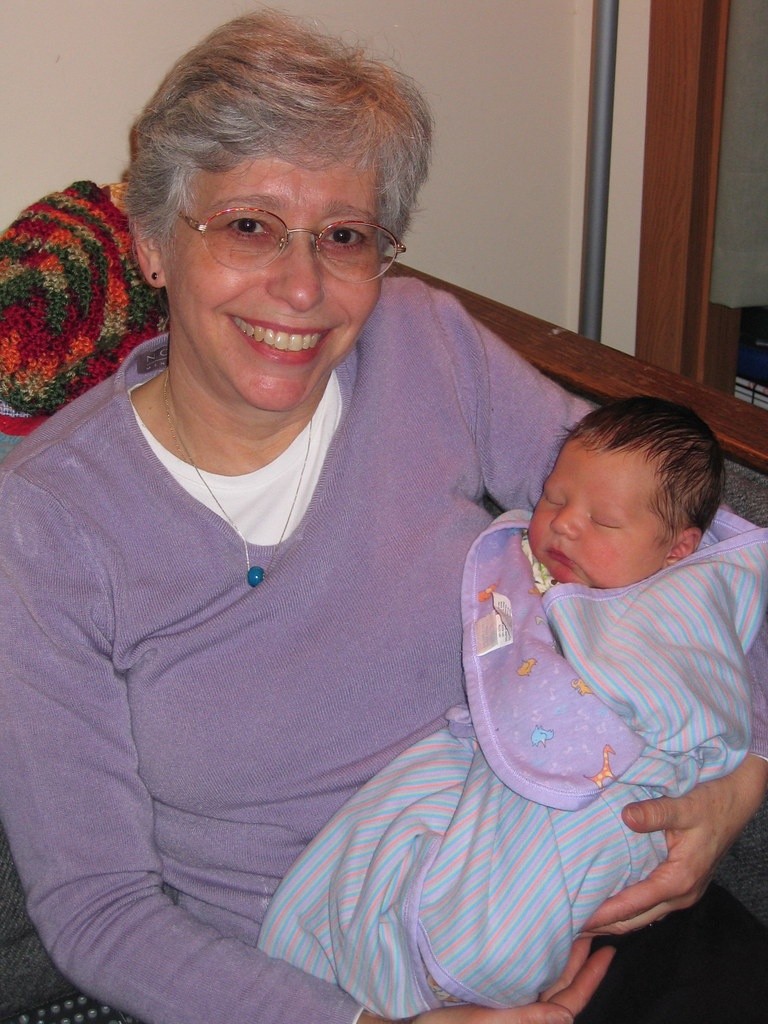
[258,397,768,1020]
[0,8,768,1024]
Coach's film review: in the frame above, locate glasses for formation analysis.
[178,207,407,283]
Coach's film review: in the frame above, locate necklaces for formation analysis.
[165,364,312,587]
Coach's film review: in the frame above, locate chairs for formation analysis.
[0,172,768,1024]
